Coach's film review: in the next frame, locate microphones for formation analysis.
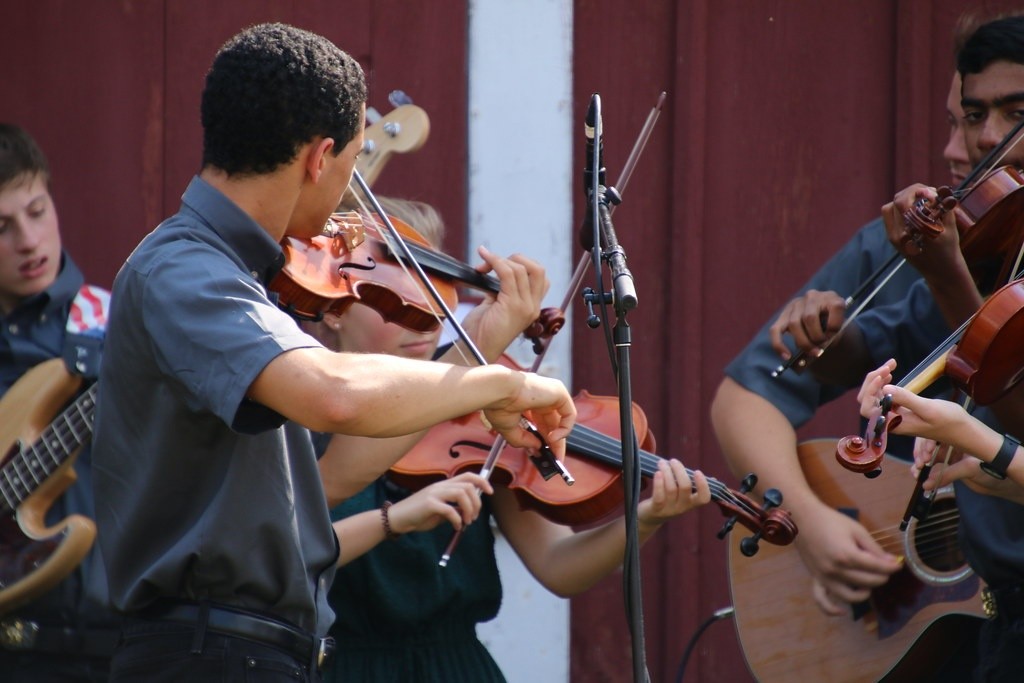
[579,104,606,252]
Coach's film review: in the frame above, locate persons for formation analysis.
[0,123,115,683]
[90,22,578,683]
[293,194,711,683]
[712,12,1024,683]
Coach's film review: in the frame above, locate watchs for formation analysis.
[980,433,1022,480]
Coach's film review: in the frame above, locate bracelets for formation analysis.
[381,500,400,540]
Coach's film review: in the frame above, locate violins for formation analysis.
[380,339,799,558]
[273,206,565,355]
[835,278,1024,479]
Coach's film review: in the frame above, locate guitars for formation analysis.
[894,163,1024,286]
[726,436,999,683]
[0,89,433,608]
